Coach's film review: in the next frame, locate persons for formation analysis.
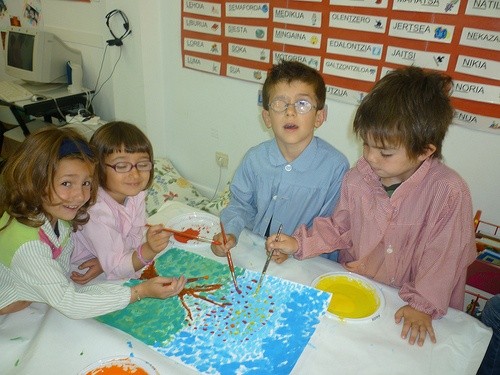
[209,61,351,264]
[0,121,188,318]
[264,65,478,347]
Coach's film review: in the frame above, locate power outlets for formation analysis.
[215,151,228,167]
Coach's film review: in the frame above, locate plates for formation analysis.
[163,212,223,251]
[76,355,159,375]
[309,272,386,323]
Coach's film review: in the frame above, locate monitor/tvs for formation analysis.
[4,26,72,92]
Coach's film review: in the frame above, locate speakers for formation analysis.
[66,62,82,92]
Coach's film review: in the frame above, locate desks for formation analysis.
[0,78,95,137]
[24,201,493,375]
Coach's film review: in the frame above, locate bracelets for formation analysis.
[133,286,140,301]
[137,244,153,266]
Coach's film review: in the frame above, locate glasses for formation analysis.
[267,100,318,114]
[103,160,154,173]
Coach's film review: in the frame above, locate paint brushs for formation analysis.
[162,277,200,286]
[146,224,222,245]
[219,222,241,293]
[254,223,283,292]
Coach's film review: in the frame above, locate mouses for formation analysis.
[31,94,46,101]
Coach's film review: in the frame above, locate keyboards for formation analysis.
[0,79,34,103]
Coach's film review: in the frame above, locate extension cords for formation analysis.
[65,113,101,126]
